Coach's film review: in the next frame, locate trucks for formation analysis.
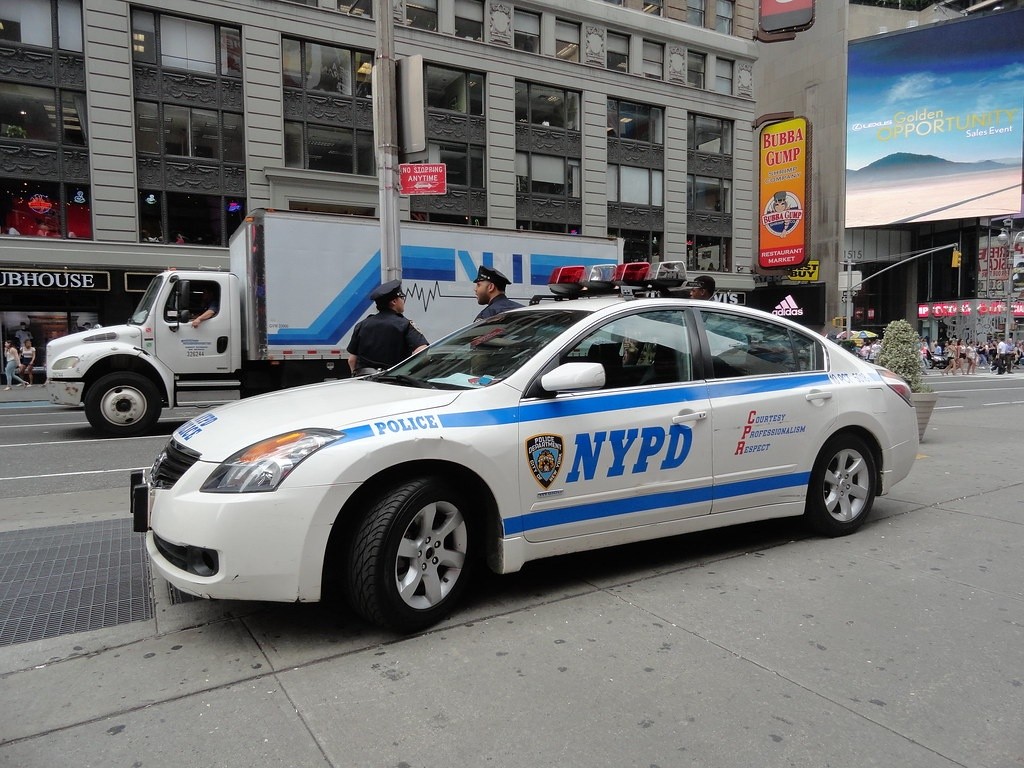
[44,207,626,438]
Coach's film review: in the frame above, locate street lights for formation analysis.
[997,228,1024,344]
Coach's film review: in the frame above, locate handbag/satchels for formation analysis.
[992,358,1001,367]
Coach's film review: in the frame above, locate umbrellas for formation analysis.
[836,330,877,346]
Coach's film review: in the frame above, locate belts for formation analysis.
[355,367,384,376]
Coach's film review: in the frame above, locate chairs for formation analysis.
[587,340,623,386]
[745,346,788,375]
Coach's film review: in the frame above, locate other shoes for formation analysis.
[17,384,23,386]
[27,384,32,387]
[978,367,985,369]
[24,382,29,389]
[40,384,46,387]
[3,387,13,390]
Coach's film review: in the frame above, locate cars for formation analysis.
[131,262,919,634]
[925,354,949,369]
[718,341,809,375]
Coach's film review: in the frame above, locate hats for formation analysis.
[473,265,512,287]
[369,279,406,305]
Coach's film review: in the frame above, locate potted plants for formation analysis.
[872,319,937,444]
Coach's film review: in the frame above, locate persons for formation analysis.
[14,322,34,359]
[911,332,1024,375]
[588,316,674,389]
[690,275,715,301]
[4,340,36,391]
[839,337,882,364]
[470,265,534,377]
[187,286,218,328]
[347,280,430,375]
[175,233,184,244]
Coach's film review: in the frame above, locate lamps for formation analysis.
[738,266,741,272]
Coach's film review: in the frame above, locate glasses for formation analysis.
[398,295,406,300]
